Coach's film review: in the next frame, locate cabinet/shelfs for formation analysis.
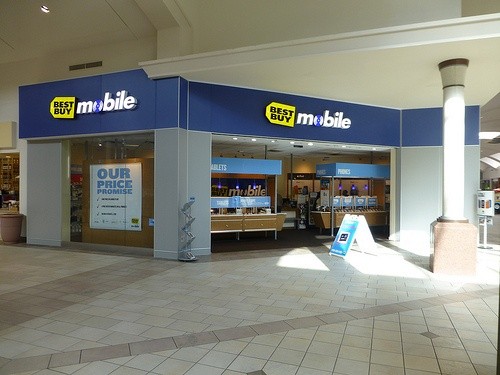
[210,214,285,232]
